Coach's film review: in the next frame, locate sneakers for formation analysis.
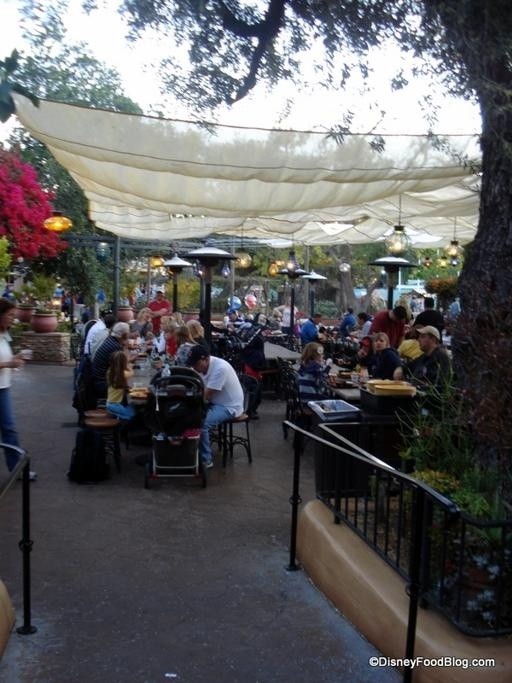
[12,471,37,480]
[203,462,214,469]
[248,412,260,419]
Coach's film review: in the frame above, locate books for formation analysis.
[307,399,362,422]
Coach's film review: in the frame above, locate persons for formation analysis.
[55,285,461,433]
[403,326,453,503]
[183,344,245,469]
[0,297,37,482]
[3,282,17,307]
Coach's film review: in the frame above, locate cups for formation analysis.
[21,349,33,359]
[14,359,24,371]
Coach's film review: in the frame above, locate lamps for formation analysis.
[97,242,110,263]
[222,219,300,279]
[387,192,464,269]
[44,210,73,235]
[338,262,352,273]
[150,255,164,268]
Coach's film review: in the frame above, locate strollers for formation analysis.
[143,365,213,490]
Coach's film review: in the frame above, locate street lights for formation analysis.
[274,265,330,333]
[182,244,240,354]
[158,250,194,313]
[370,254,419,314]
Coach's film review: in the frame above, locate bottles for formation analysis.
[162,364,171,378]
[350,368,359,383]
[360,367,369,382]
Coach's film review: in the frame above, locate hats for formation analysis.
[416,325,441,340]
[111,322,131,339]
[184,346,206,365]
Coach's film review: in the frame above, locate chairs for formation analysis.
[75,332,364,468]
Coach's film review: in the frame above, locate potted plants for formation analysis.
[98,271,114,327]
[180,279,201,323]
[116,271,137,323]
[13,285,33,323]
[30,272,58,333]
